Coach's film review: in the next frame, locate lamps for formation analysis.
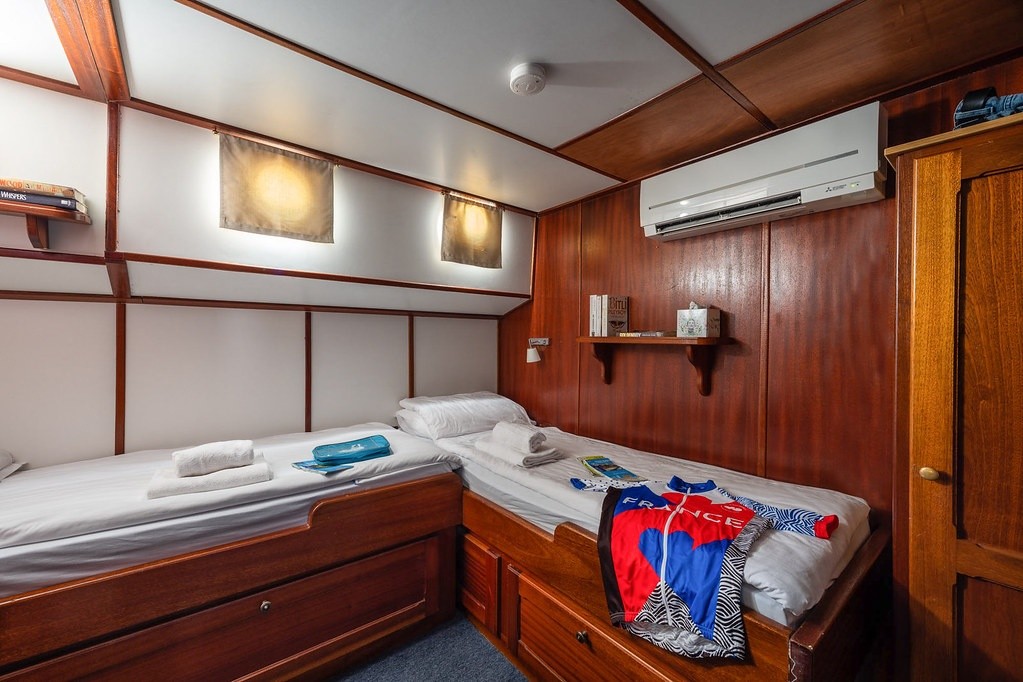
[526,339,542,363]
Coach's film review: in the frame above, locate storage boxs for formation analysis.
[676,305,721,338]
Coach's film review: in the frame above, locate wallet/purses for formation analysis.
[312,435,391,465]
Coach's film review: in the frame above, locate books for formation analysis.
[620,332,677,337]
[0,178,88,215]
[590,295,627,337]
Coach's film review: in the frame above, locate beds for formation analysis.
[395,428,892,682]
[0,420,465,681]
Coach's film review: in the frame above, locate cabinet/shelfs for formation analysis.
[456,524,692,682]
[0,536,443,682]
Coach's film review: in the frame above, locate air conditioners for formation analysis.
[639,101,889,242]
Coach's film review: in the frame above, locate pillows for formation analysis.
[394,409,436,439]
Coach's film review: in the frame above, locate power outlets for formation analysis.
[529,337,549,346]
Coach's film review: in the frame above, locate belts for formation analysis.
[959,87,998,125]
